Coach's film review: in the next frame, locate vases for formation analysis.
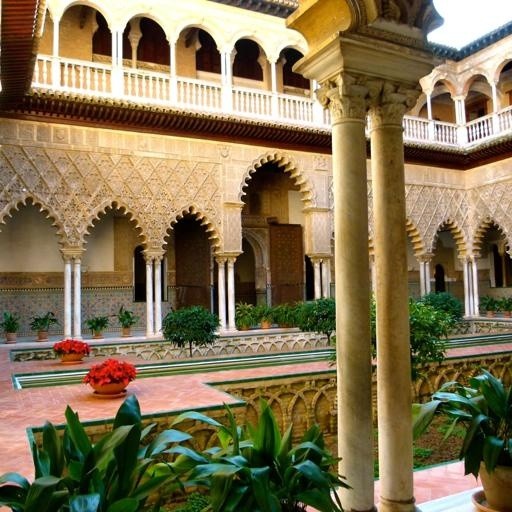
[90,379,128,398]
[60,353,84,365]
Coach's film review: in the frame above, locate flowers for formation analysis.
[53,339,90,355]
[82,357,136,386]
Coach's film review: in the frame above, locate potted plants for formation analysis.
[118,306,139,337]
[1,312,19,344]
[480,295,512,318]
[29,311,57,342]
[85,317,109,339]
[411,369,512,512]
[236,301,294,331]
